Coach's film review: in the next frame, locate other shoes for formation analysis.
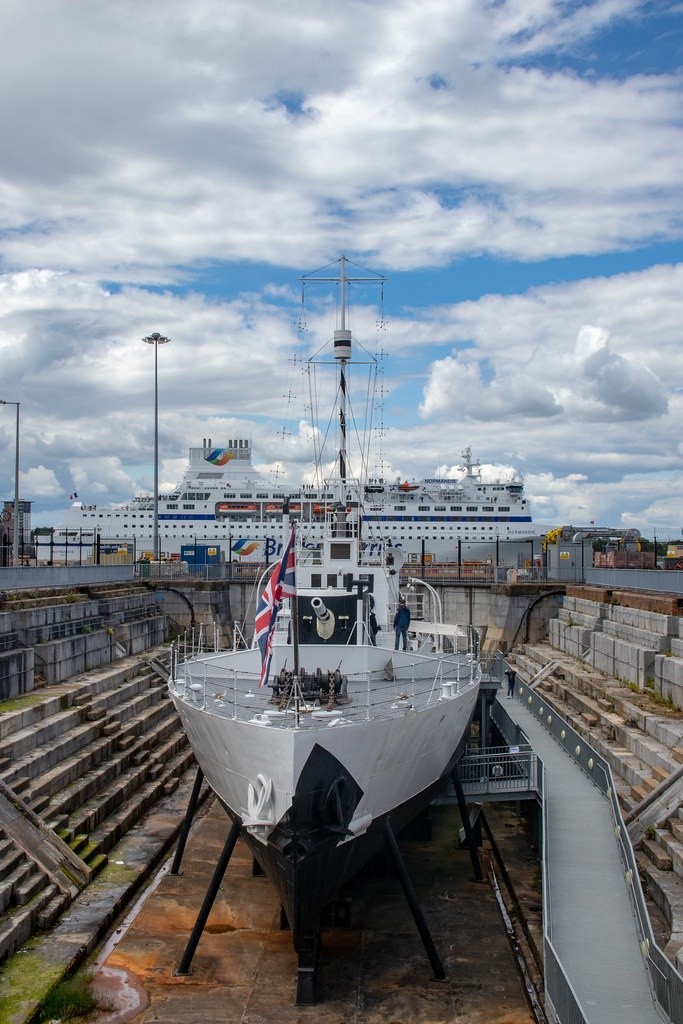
[394,647,398,650]
[507,695,513,699]
[403,647,407,651]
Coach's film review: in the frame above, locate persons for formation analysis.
[505,668,517,700]
[392,600,410,652]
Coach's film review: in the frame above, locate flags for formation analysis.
[69,492,79,501]
[254,527,295,690]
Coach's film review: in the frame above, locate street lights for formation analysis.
[141,332,171,575]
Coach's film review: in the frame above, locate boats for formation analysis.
[166,619,482,956]
[29,462,541,568]
